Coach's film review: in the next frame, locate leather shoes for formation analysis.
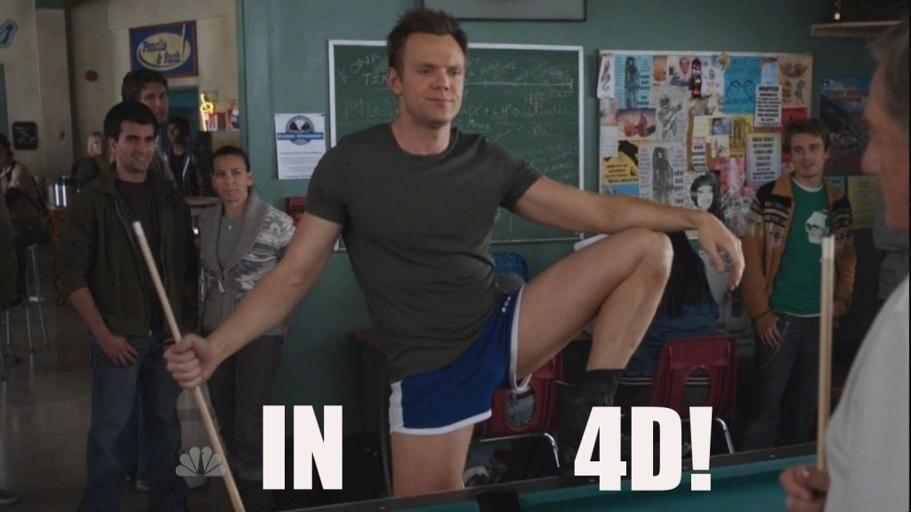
[563,416,692,470]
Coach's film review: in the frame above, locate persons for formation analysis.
[161,8,746,498]
[691,175,723,221]
[195,144,299,512]
[739,118,858,450]
[72,132,104,194]
[778,18,909,512]
[162,117,209,196]
[59,102,201,512]
[670,57,692,85]
[121,69,211,492]
[0,133,51,305]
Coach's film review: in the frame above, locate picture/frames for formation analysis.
[128,21,198,78]
[421,0,587,22]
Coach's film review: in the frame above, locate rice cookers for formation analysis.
[48,177,77,208]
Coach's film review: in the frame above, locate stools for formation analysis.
[5,243,49,352]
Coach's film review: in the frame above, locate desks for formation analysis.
[286,443,816,512]
[349,331,590,495]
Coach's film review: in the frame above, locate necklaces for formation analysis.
[225,217,237,231]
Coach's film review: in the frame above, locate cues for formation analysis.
[818,235,836,477]
[134,222,252,512]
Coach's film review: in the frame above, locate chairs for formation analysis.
[473,351,563,467]
[645,336,736,455]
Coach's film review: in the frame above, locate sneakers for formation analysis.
[529,433,560,475]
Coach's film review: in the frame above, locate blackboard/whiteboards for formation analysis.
[327,38,585,253]
